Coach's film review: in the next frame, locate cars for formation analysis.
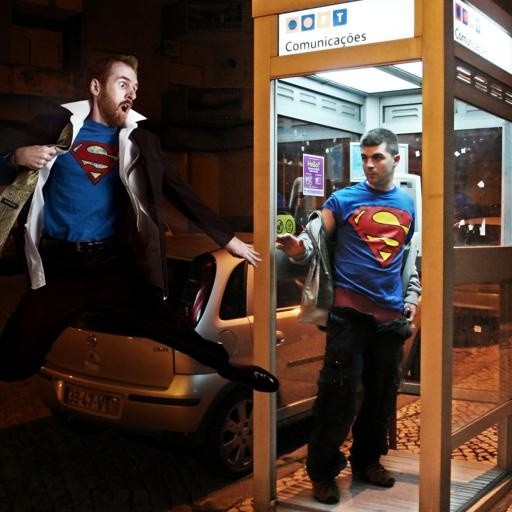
[36,231,421,484]
[452,215,502,345]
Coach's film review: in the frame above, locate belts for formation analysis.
[51,238,119,251]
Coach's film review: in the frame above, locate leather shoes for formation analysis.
[223,363,279,393]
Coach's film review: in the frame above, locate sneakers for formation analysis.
[352,461,395,487]
[311,479,340,504]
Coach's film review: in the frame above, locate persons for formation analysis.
[0,47,282,394]
[289,144,332,226]
[277,127,423,504]
